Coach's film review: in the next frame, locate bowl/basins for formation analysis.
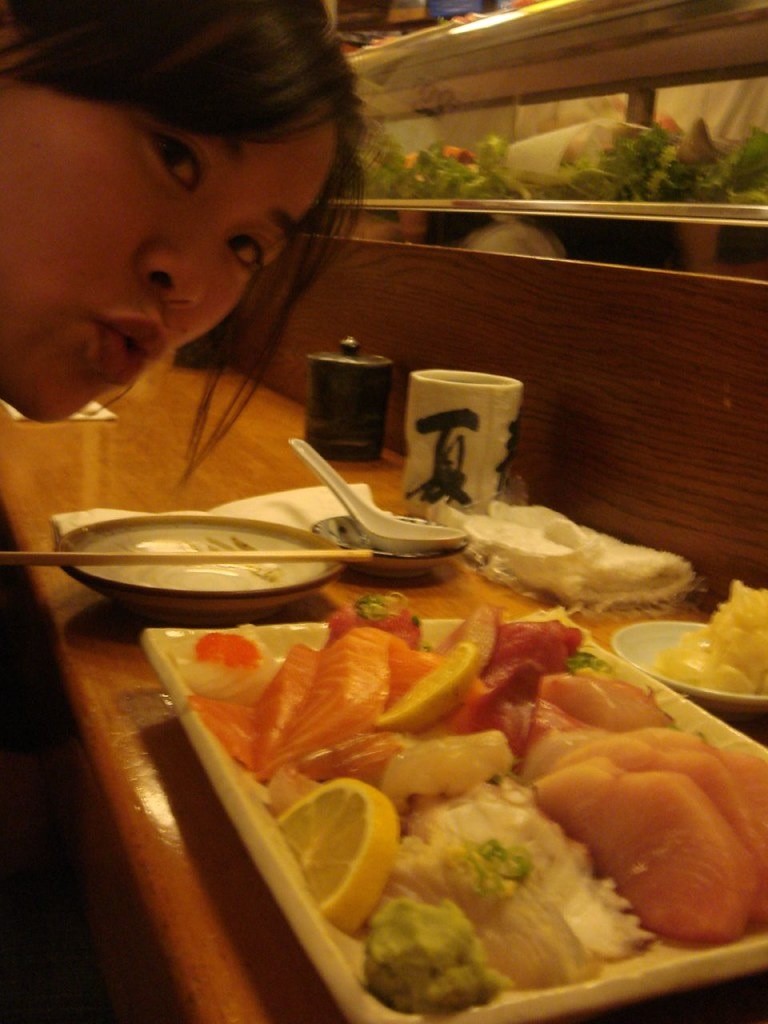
[56,514,349,631]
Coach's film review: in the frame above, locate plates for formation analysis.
[146,614,768,1024]
[313,516,466,577]
[612,622,768,721]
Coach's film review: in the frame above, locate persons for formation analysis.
[0,1,339,426]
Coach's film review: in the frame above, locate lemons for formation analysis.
[280,777,401,931]
[374,641,479,731]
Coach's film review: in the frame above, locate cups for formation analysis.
[403,370,524,522]
[305,339,394,462]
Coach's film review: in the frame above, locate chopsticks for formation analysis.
[0,548,372,565]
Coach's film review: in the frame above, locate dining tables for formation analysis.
[0,353,767,1024]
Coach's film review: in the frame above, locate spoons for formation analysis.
[288,438,467,552]
